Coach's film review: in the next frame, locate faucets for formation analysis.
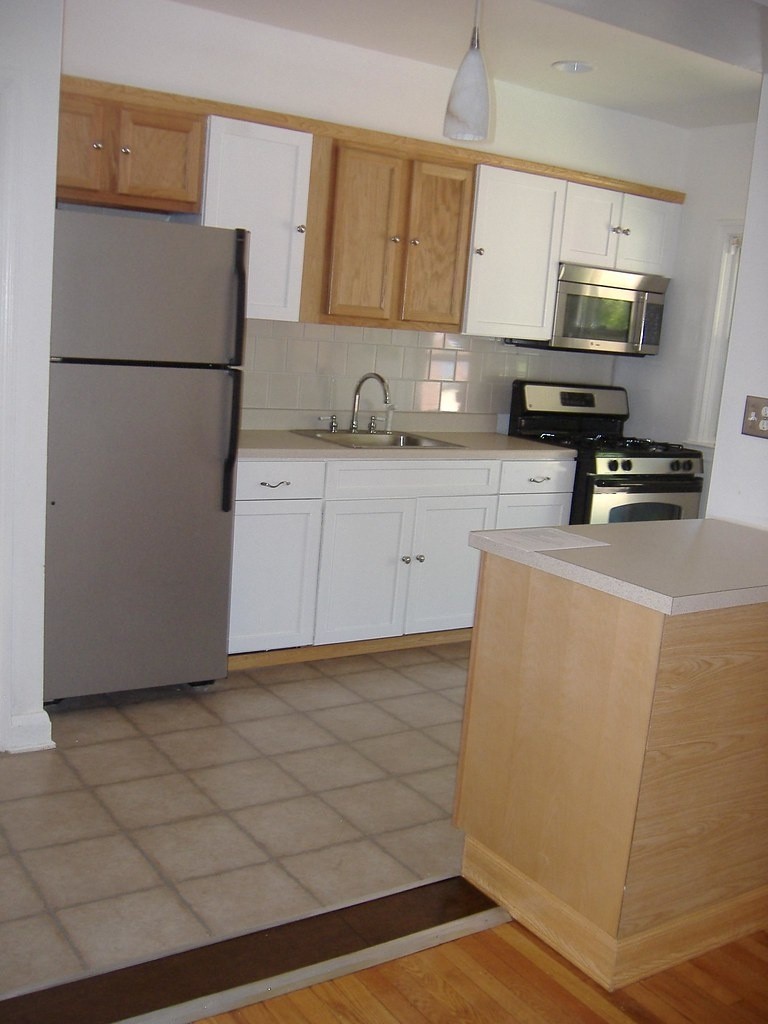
[350,372,392,431]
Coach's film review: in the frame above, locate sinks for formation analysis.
[288,429,468,450]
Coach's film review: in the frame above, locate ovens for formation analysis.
[569,477,703,524]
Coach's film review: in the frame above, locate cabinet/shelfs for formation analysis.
[461,163,568,341]
[300,121,477,335]
[56,90,207,215]
[560,183,686,279]
[228,458,326,654]
[201,115,314,323]
[312,460,499,646]
[496,457,577,529]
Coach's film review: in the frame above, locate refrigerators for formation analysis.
[43,210,250,701]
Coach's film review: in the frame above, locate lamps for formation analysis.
[442,0,490,142]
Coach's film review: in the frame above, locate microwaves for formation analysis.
[549,262,670,354]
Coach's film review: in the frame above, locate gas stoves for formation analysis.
[509,381,704,476]
